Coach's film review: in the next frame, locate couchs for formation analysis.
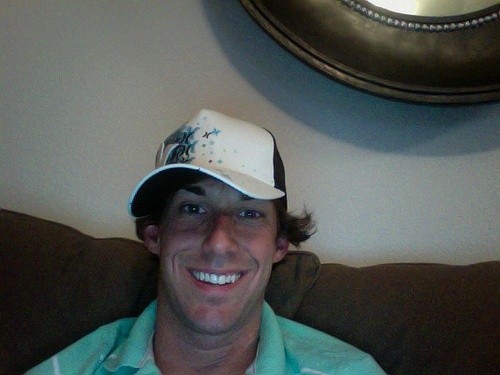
[0,209,500,375]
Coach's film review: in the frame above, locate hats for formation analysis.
[127,107,287,219]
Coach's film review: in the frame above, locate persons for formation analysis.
[23,108,388,375]
